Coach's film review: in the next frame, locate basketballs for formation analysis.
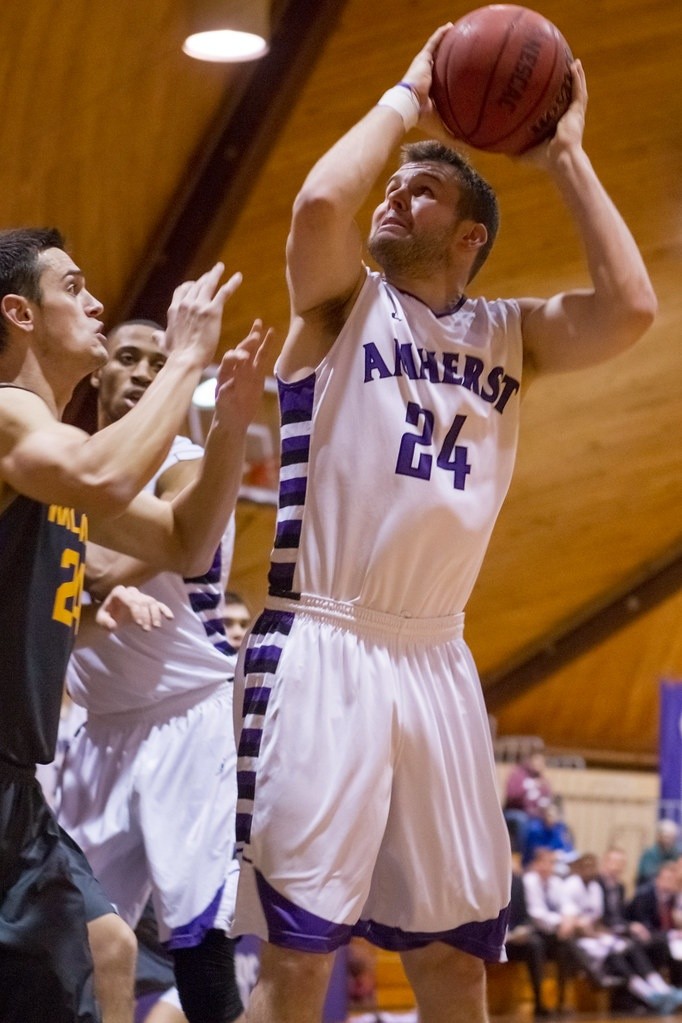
[429,3,575,155]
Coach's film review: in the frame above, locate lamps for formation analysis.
[180,0,271,66]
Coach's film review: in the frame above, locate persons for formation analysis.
[0,228,276,1023]
[224,590,251,646]
[244,22,659,1023]
[41,319,249,1023]
[501,742,682,1023]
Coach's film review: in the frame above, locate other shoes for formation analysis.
[659,987,682,1015]
